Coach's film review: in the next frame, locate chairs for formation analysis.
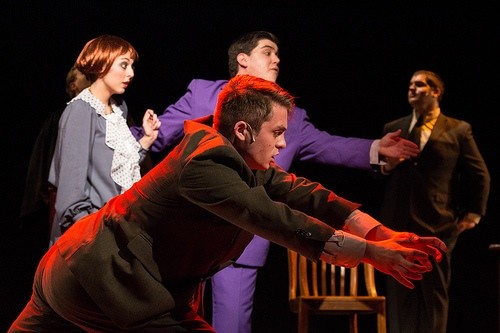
[288,248,387,333]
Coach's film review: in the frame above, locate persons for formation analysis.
[374,71,491,333]
[48,34,162,249]
[5,74,447,333]
[129,31,419,333]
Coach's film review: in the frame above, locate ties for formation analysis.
[407,114,424,152]
